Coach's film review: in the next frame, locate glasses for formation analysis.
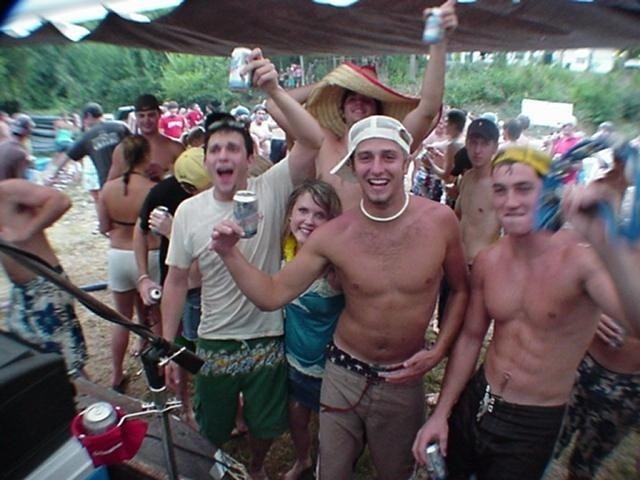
[207,118,246,131]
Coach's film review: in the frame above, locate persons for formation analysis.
[410,143,639,479]
[550,141,640,480]
[412,106,635,231]
[155,46,325,480]
[262,1,460,215]
[205,116,472,478]
[284,181,346,477]
[1,64,301,392]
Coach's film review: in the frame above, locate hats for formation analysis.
[466,118,498,141]
[133,93,160,111]
[305,61,442,152]
[327,114,414,175]
[10,114,35,136]
[173,146,213,196]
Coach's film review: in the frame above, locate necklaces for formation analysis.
[357,194,414,224]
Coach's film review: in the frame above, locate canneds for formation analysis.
[228,46,252,94]
[148,287,161,300]
[152,206,168,237]
[421,7,445,45]
[81,401,118,436]
[232,190,258,238]
[424,441,446,480]
